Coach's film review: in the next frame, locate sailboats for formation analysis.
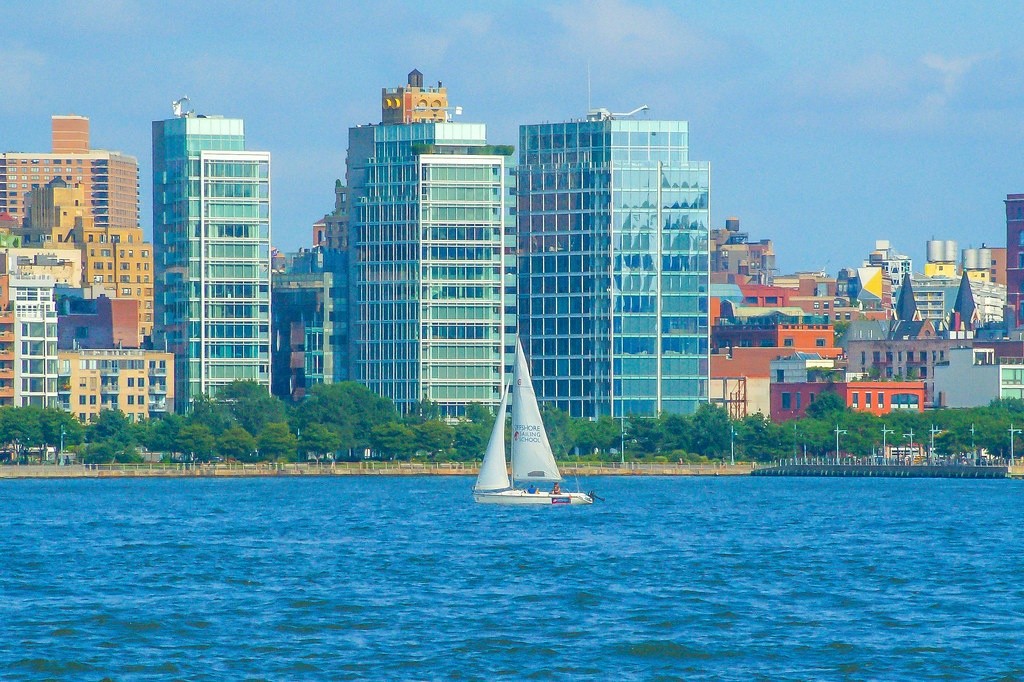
[472,333,594,504]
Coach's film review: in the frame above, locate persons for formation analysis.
[528,485,540,494]
[551,482,562,495]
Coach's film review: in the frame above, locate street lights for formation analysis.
[732,426,738,466]
[902,428,915,467]
[60,423,67,465]
[881,424,895,460]
[930,424,942,465]
[834,424,847,465]
[622,431,628,463]
[1007,424,1022,466]
